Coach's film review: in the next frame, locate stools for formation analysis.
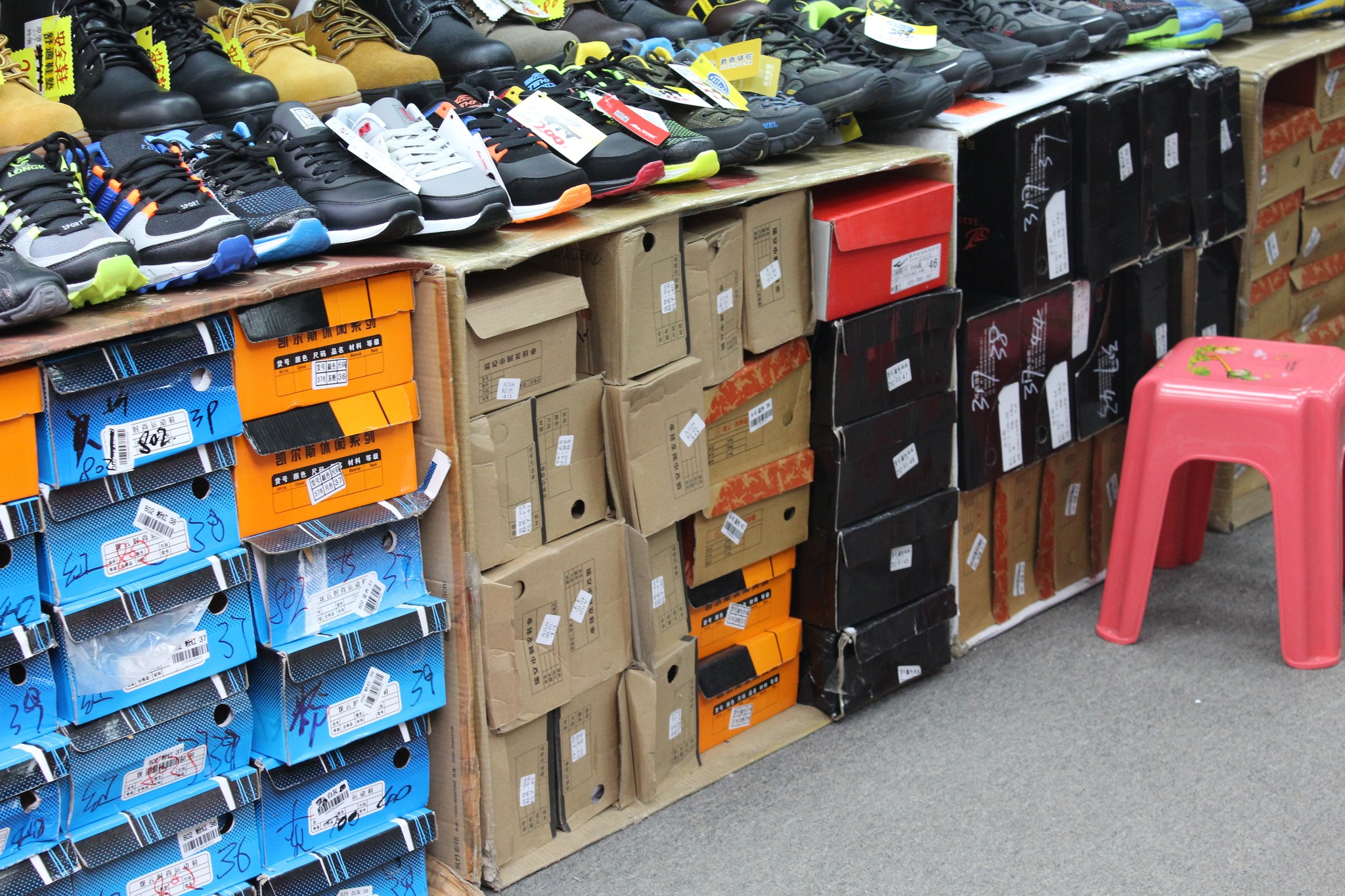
[1097,337,1345,671]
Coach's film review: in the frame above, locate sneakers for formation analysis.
[245,101,425,254]
[0,131,149,309]
[718,10,892,123]
[62,131,260,293]
[1139,0,1224,49]
[1028,0,1129,52]
[457,1,795,78]
[958,0,1091,65]
[851,0,993,97]
[622,37,829,158]
[893,0,1046,88]
[331,97,512,245]
[1188,0,1252,36]
[391,83,592,223]
[793,1,955,132]
[522,64,720,185]
[0,236,71,328]
[465,67,665,200]
[1082,0,1180,46]
[561,40,768,168]
[1237,0,1345,26]
[144,121,330,263]
[0,1,517,152]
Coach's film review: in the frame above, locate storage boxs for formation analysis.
[0,20,1345,896]
